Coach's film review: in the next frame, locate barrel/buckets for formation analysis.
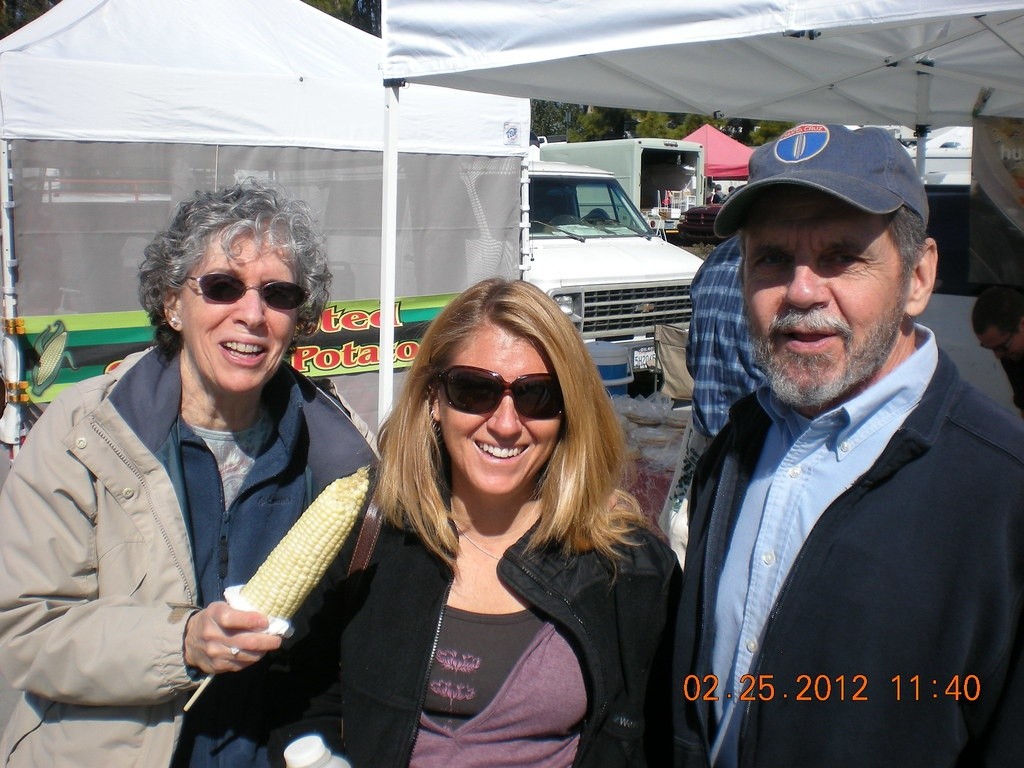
[585,345,635,401]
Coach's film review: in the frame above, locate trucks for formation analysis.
[530,138,706,231]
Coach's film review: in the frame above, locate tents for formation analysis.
[379,0,1024,432]
[842,125,974,184]
[0,0,532,466]
[682,124,754,181]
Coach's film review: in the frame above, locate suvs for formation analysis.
[525,161,706,342]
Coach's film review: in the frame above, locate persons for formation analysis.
[269,277,683,768]
[972,288,1024,416]
[675,123,1024,768]
[657,235,770,568]
[0,177,381,767]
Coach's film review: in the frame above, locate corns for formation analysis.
[239,464,369,621]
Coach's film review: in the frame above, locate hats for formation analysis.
[713,123,930,241]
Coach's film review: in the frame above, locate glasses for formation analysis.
[186,272,311,311]
[436,364,564,420]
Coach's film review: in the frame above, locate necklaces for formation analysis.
[455,519,503,563]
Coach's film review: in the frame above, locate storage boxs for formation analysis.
[659,208,682,220]
